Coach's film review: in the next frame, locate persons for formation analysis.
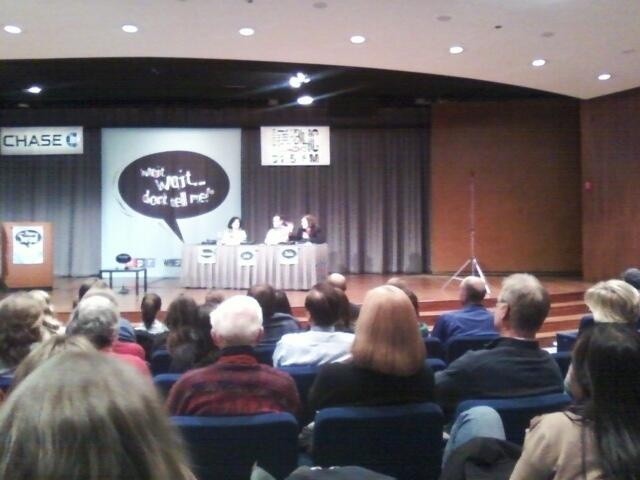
[0,270,640,414]
[508,325,638,479]
[264,216,288,244]
[295,214,323,242]
[0,349,199,479]
[226,217,247,245]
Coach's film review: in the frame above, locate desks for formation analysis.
[100,267,148,297]
[179,241,330,291]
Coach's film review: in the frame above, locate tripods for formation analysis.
[441,178,491,295]
